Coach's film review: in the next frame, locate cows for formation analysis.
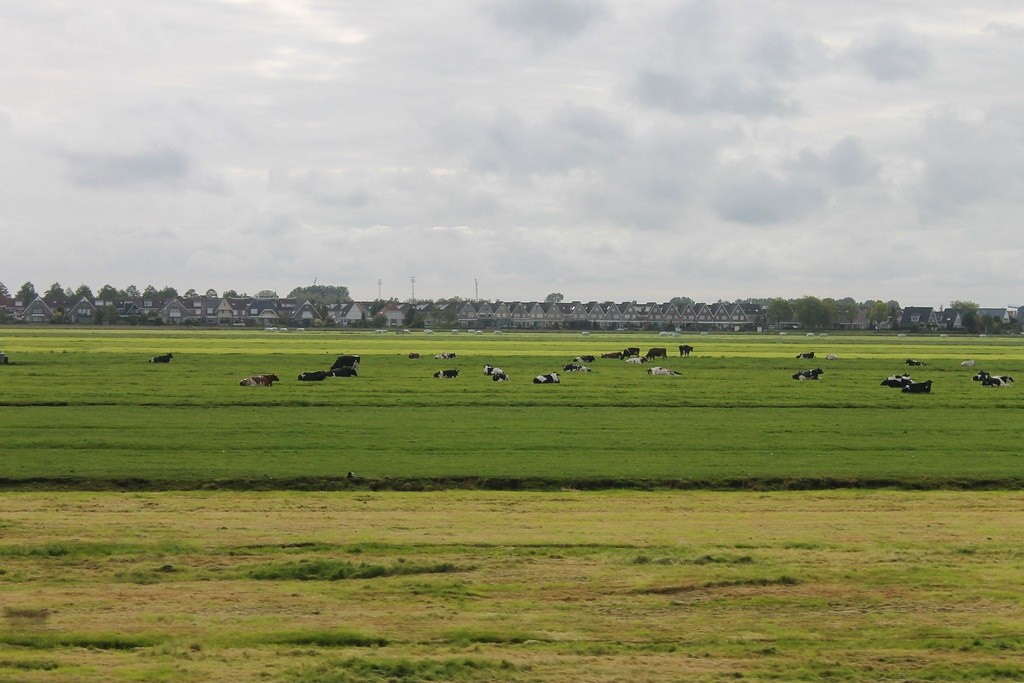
[409,353,423,359]
[647,367,677,375]
[625,357,649,364]
[573,355,596,362]
[679,345,694,357]
[533,372,560,384]
[880,358,933,394]
[435,353,456,359]
[563,364,591,372]
[972,371,1014,387]
[298,355,360,382]
[434,369,460,378]
[149,353,173,363]
[645,348,667,360]
[601,351,623,358]
[792,352,824,380]
[484,364,504,375]
[493,374,510,381]
[240,372,280,387]
[620,348,640,360]
